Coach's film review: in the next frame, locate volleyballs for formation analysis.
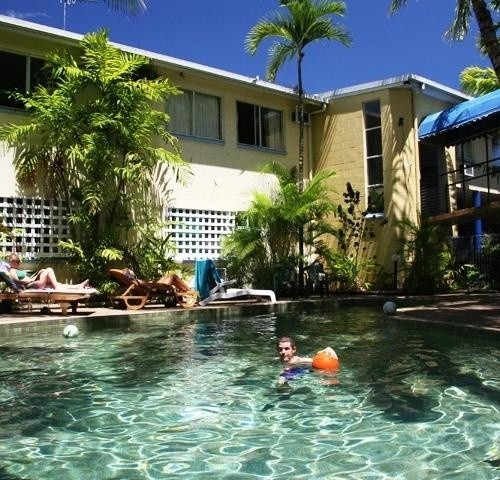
[312,352,340,371]
[63,324,80,338]
[382,301,397,315]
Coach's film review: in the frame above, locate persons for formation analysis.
[273,337,313,386]
[123,268,191,292]
[5,254,89,290]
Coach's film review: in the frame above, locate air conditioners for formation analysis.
[291,109,311,125]
[461,164,475,178]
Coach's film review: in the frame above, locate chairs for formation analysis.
[0,258,100,318]
[104,268,202,311]
[192,257,279,306]
